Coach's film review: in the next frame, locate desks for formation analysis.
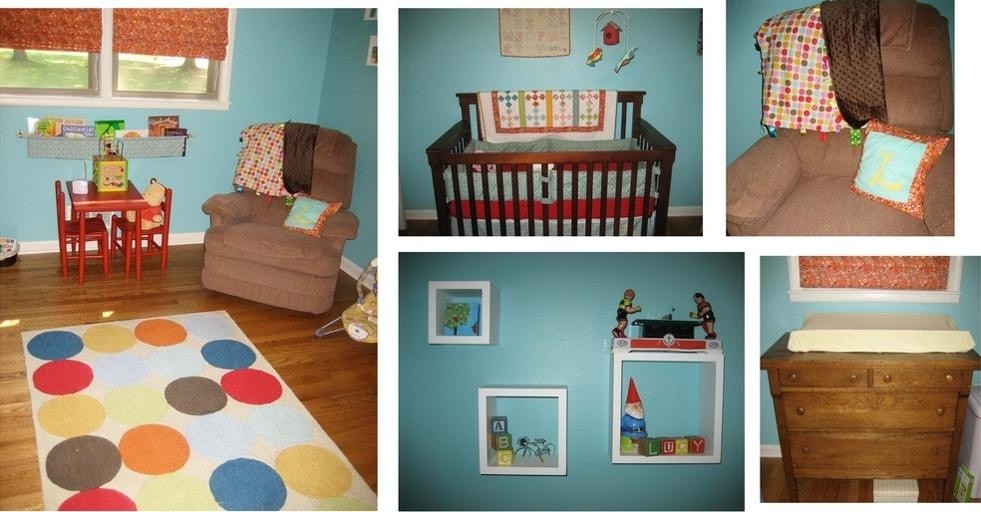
[66,179,146,284]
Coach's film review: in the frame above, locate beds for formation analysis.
[425,91,676,236]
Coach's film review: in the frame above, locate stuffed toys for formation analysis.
[126,178,166,231]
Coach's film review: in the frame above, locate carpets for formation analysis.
[19,309,378,510]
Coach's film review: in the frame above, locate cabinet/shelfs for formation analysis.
[760,332,981,501]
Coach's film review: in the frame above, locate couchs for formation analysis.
[202,124,359,316]
[726,3,955,236]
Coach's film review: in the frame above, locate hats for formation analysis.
[625,376,642,405]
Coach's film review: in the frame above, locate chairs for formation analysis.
[55,181,110,280]
[111,178,172,279]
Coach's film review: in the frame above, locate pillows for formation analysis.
[850,118,950,220]
[282,191,343,238]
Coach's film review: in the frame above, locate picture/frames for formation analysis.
[477,385,568,476]
[785,257,964,305]
[427,279,492,347]
[613,352,725,465]
